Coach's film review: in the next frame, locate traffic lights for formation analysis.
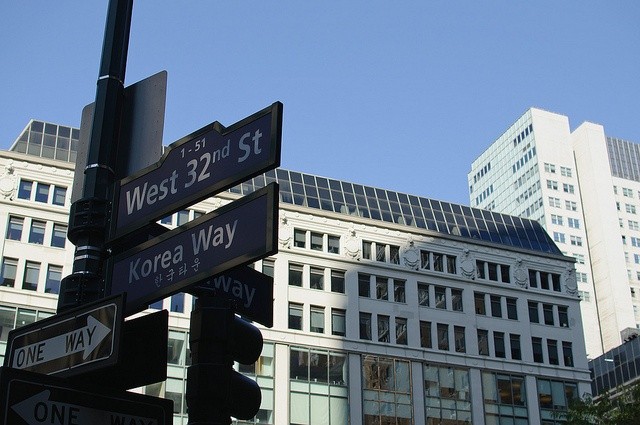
[186,297,263,425]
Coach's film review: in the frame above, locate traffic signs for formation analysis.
[100,100,282,242]
[117,309,169,392]
[105,183,280,315]
[0,367,176,425]
[126,223,275,330]
[4,292,129,380]
